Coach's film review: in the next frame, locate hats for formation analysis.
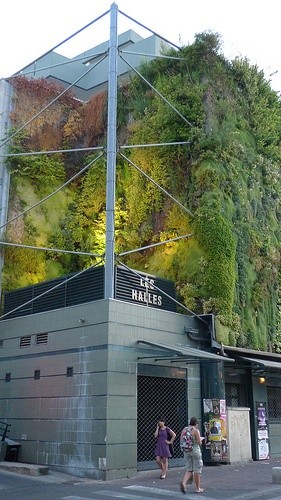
[187,416,197,425]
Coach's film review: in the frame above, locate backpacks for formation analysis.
[180,427,195,452]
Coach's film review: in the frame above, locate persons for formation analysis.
[179,416,204,495]
[213,446,220,455]
[210,421,219,434]
[155,418,176,479]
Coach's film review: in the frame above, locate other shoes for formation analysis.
[160,475,166,478]
[195,488,204,492]
[180,481,186,495]
